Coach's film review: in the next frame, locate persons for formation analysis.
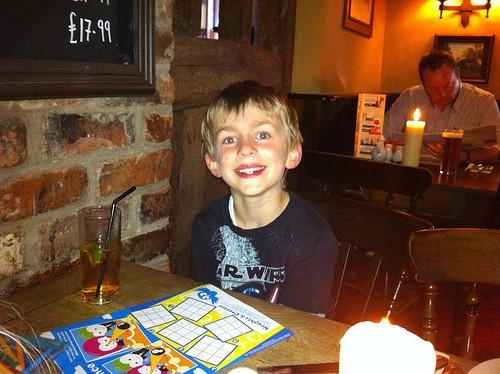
[190,81,338,319]
[382,54,500,166]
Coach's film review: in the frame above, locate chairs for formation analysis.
[287,148,500,361]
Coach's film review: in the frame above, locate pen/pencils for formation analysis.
[16,338,25,370]
[21,346,55,374]
[0,335,18,367]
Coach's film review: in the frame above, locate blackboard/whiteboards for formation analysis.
[0,0,156,102]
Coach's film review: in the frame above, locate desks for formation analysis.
[347,140,500,213]
[0,262,482,374]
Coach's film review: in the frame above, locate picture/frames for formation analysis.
[434,34,493,83]
[343,0,375,38]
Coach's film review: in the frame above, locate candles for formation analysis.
[338,317,436,374]
[402,108,426,167]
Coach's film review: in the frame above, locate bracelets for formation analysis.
[461,150,470,166]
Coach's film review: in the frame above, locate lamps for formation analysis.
[437,0,492,27]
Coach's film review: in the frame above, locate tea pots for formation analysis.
[371,137,387,162]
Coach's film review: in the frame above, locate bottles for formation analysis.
[384,143,393,162]
[393,146,404,163]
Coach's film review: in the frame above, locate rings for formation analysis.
[434,152,437,155]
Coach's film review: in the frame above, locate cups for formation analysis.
[439,128,464,175]
[338,321,436,374]
[77,206,122,305]
